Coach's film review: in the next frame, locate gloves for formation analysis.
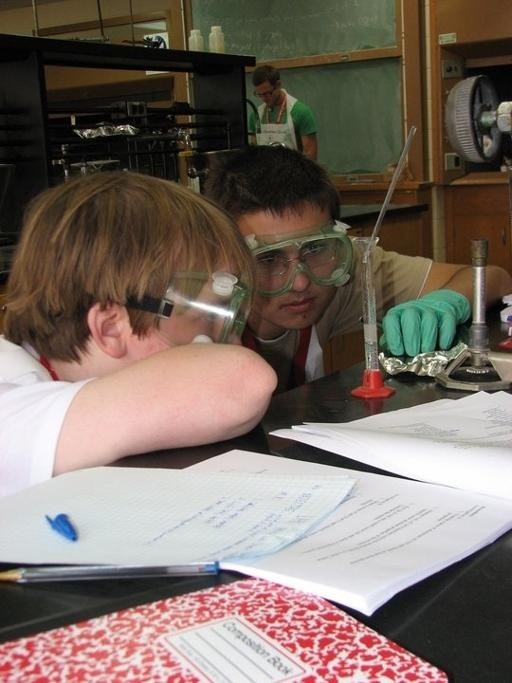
[378,288,472,355]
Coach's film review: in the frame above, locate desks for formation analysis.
[2,309,512,679]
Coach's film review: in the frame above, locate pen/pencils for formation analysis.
[0,560,221,584]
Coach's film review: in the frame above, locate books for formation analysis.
[0,576,449,683]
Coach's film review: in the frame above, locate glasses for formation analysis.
[251,87,276,97]
[248,222,354,297]
[111,268,252,351]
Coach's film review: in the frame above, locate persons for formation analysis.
[0,170,278,493]
[247,65,319,161]
[205,145,512,392]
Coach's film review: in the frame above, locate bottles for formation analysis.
[187,26,225,52]
[352,236,380,371]
